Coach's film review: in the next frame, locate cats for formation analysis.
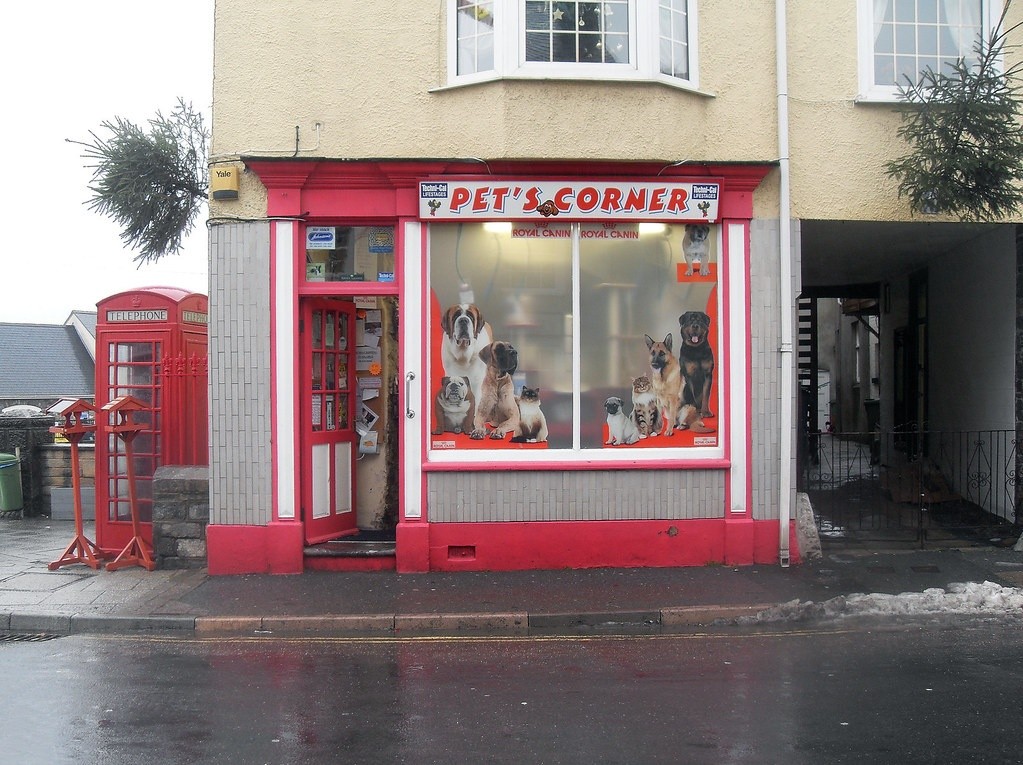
[631,372,664,439]
[515,385,548,442]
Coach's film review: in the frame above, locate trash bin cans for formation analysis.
[0,453,24,512]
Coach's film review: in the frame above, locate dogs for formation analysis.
[604,396,640,445]
[644,310,715,437]
[433,303,520,443]
